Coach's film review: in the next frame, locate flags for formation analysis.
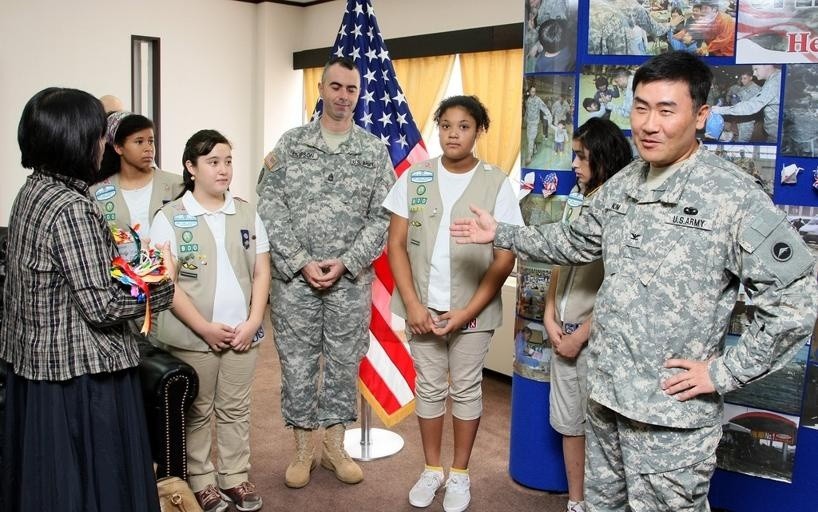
[309,0,430,430]
[422,55,462,158]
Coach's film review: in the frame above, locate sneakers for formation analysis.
[408,468,446,509]
[218,479,264,511]
[442,471,472,512]
[194,485,229,512]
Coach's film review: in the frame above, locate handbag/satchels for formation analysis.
[155,475,204,512]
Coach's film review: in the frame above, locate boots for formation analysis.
[320,421,366,486]
[284,425,319,489]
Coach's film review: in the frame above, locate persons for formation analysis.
[1,88,180,512]
[543,117,632,512]
[83,93,272,512]
[448,50,816,512]
[381,95,522,512]
[523,1,783,205]
[512,269,554,380]
[255,56,400,489]
